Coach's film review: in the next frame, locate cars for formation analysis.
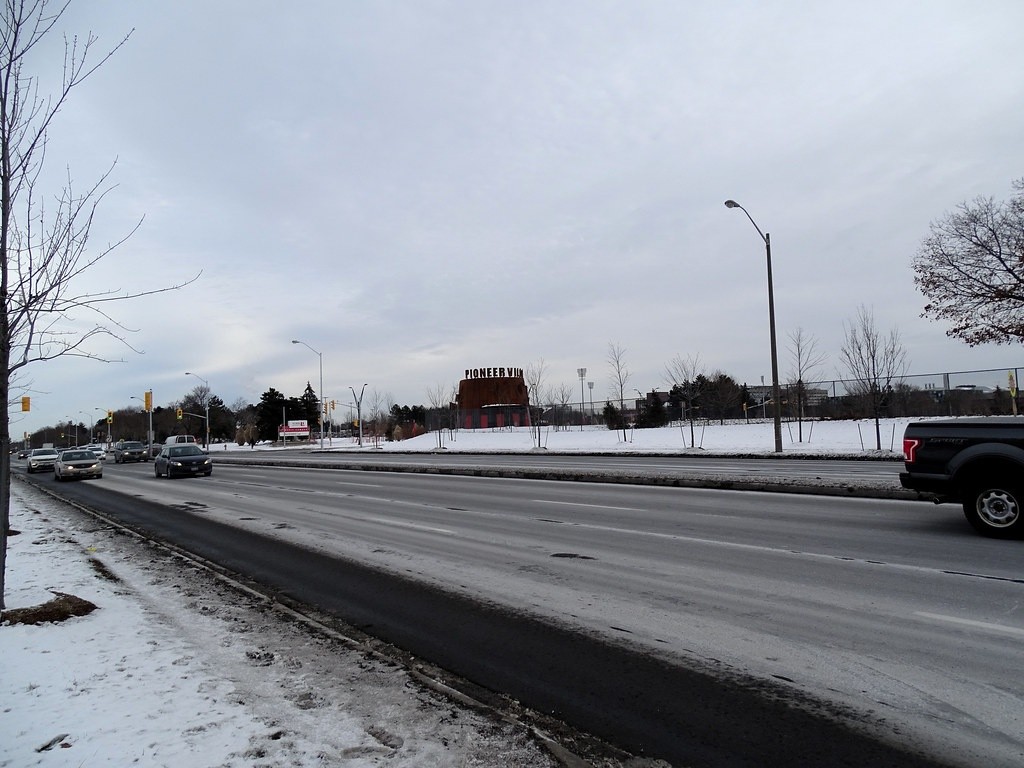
[113,440,149,464]
[53,449,103,482]
[17,444,107,461]
[154,446,212,480]
[144,443,163,457]
[25,448,61,474]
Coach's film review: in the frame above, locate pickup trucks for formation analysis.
[897,416,1024,540]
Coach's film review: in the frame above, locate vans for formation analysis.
[163,434,198,448]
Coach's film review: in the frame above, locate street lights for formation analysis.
[681,402,700,421]
[292,340,323,451]
[762,398,788,419]
[724,199,783,452]
[95,408,111,455]
[58,415,78,448]
[79,410,93,445]
[130,396,153,458]
[185,372,209,451]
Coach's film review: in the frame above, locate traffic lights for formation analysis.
[107,410,113,424]
[324,402,328,415]
[177,410,182,420]
[331,399,335,410]
